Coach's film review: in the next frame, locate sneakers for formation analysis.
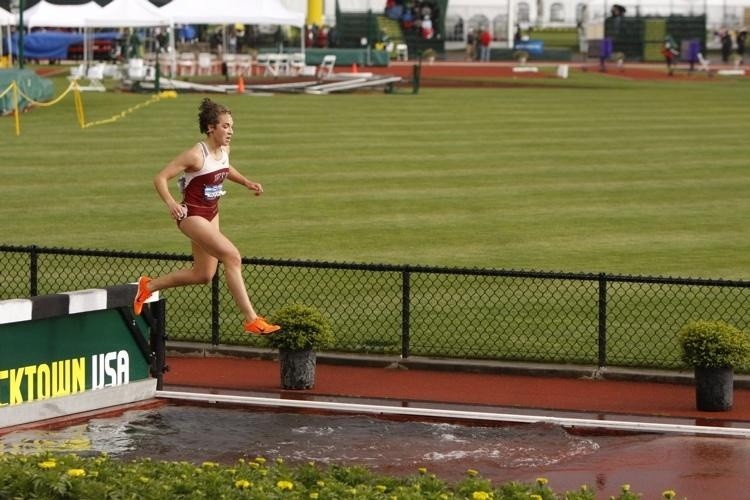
[133,276,154,315]
[243,316,282,336]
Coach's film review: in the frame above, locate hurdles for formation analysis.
[0,280,166,429]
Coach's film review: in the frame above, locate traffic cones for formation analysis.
[236,74,246,94]
[351,63,358,74]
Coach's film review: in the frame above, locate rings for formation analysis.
[177,211,180,218]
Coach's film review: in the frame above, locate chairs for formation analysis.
[143,50,337,80]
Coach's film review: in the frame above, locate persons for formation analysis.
[131,97,282,334]
[118,18,750,81]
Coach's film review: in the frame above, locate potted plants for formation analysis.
[262,303,337,390]
[677,320,750,412]
